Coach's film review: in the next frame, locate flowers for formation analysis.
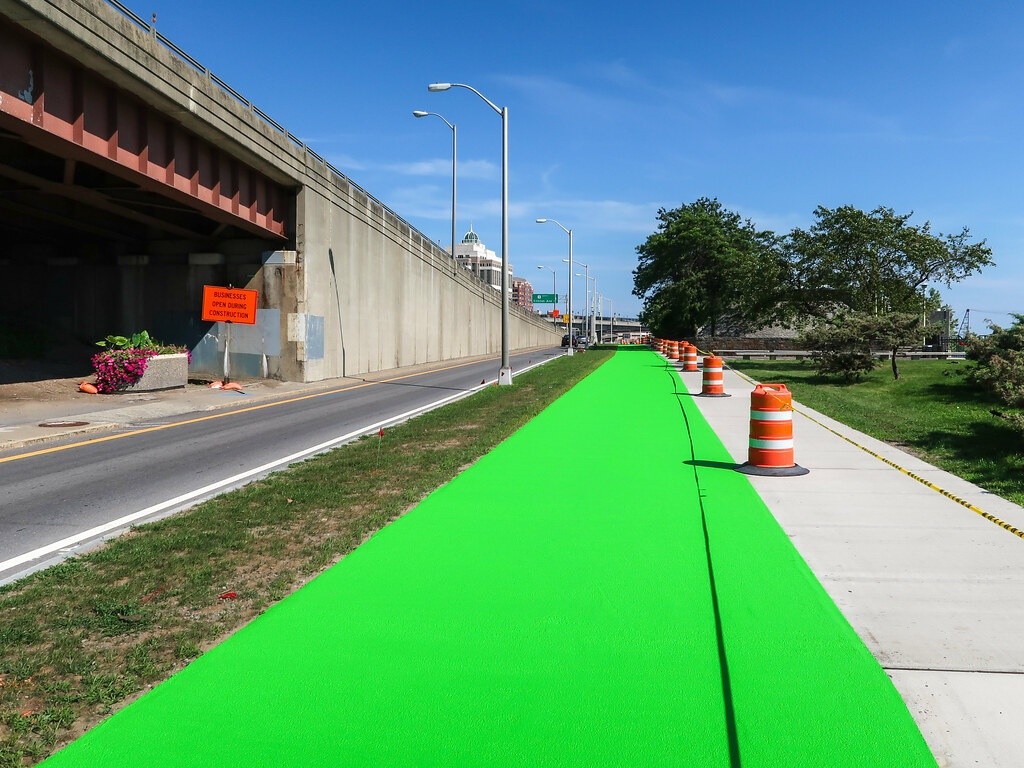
[90,329,192,394]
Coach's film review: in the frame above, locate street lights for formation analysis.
[588,290,603,345]
[428,81,513,385]
[537,265,557,331]
[563,258,589,348]
[602,296,613,343]
[413,108,458,260]
[575,273,596,344]
[535,219,574,356]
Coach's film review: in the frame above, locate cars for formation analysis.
[561,327,619,347]
